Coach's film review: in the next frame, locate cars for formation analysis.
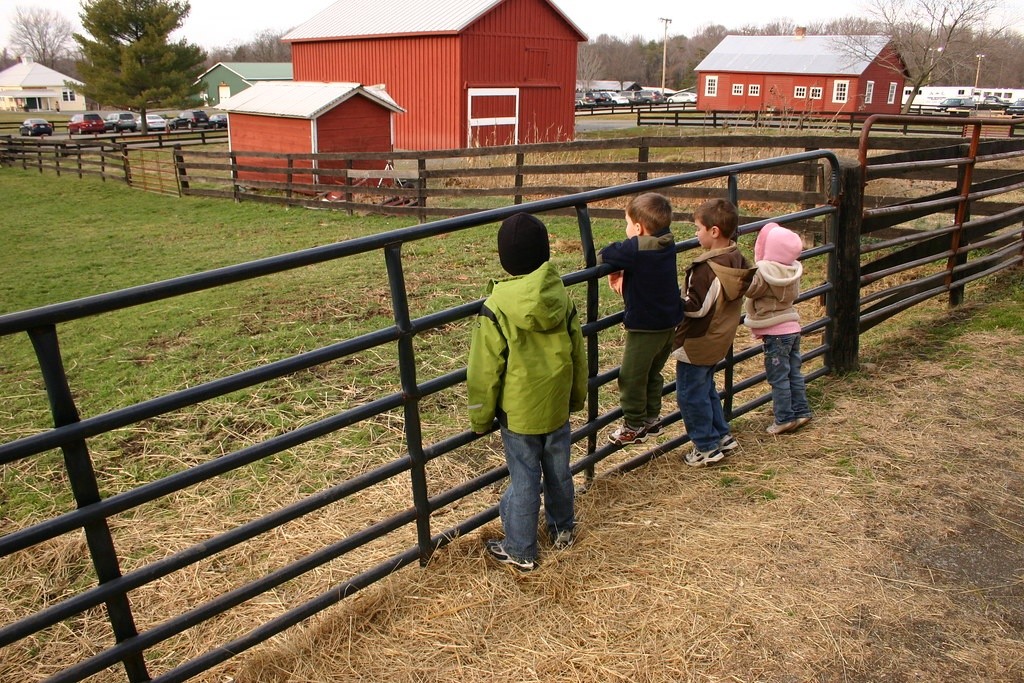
[207,112,228,129]
[1004,98,1024,116]
[19,118,53,137]
[574,89,677,111]
[667,92,697,104]
[939,97,977,118]
[135,114,167,131]
[974,95,1015,113]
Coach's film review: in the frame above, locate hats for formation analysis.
[498,212,550,275]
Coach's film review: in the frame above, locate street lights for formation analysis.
[924,46,943,85]
[974,54,985,87]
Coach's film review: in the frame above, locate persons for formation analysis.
[744,222,813,433]
[674,199,758,466]
[55,101,60,112]
[597,193,687,444]
[466,213,587,569]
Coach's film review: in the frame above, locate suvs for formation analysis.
[103,112,137,133]
[168,109,210,131]
[67,113,106,135]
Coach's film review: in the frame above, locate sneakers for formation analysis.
[765,420,795,434]
[685,447,724,466]
[786,417,810,432]
[643,417,665,437]
[717,433,738,452]
[486,538,534,571]
[553,529,573,549]
[607,424,648,445]
